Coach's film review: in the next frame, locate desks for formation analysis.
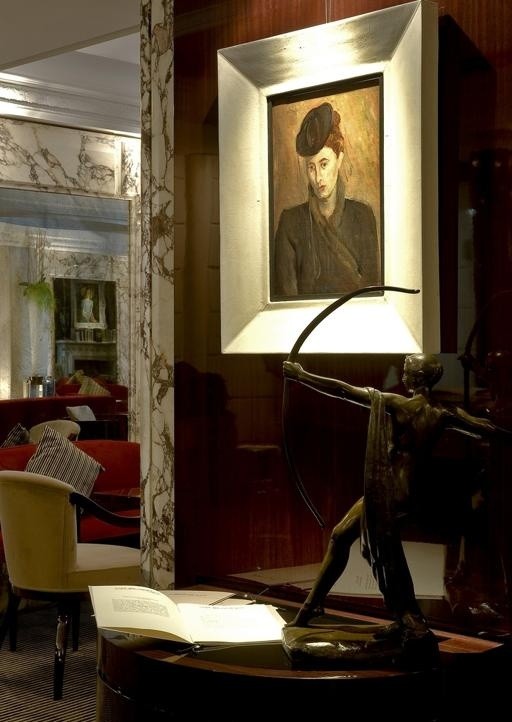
[97,563,510,720]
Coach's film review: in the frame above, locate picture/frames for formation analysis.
[70,281,108,329]
[216,1,441,358]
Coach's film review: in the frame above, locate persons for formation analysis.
[268,103,379,295]
[79,285,100,324]
[286,351,512,631]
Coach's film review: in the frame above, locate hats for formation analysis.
[295,102,335,157]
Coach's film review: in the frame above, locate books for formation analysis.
[86,581,287,648]
[328,534,451,605]
